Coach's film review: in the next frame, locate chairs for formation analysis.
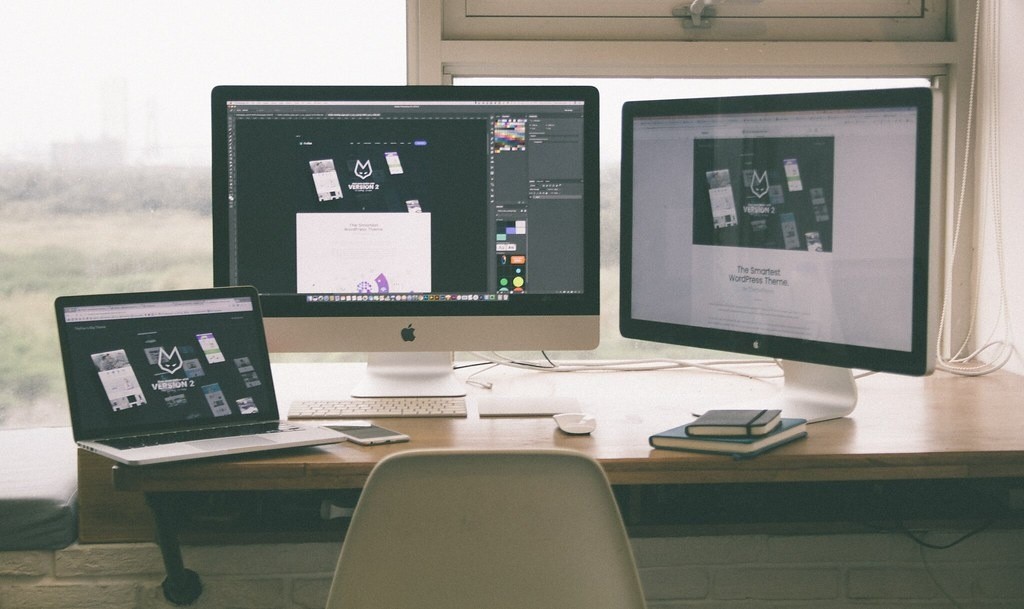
[322,448,647,609]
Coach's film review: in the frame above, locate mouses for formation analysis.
[553,412,596,434]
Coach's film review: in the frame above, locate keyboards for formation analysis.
[289,397,467,418]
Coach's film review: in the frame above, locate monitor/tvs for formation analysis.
[619,86,936,379]
[212,85,600,352]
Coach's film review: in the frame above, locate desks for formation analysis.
[74,346,1024,608]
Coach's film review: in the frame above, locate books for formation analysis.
[649,409,808,457]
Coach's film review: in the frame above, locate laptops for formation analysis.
[54,285,347,467]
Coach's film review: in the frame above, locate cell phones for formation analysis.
[318,424,410,446]
